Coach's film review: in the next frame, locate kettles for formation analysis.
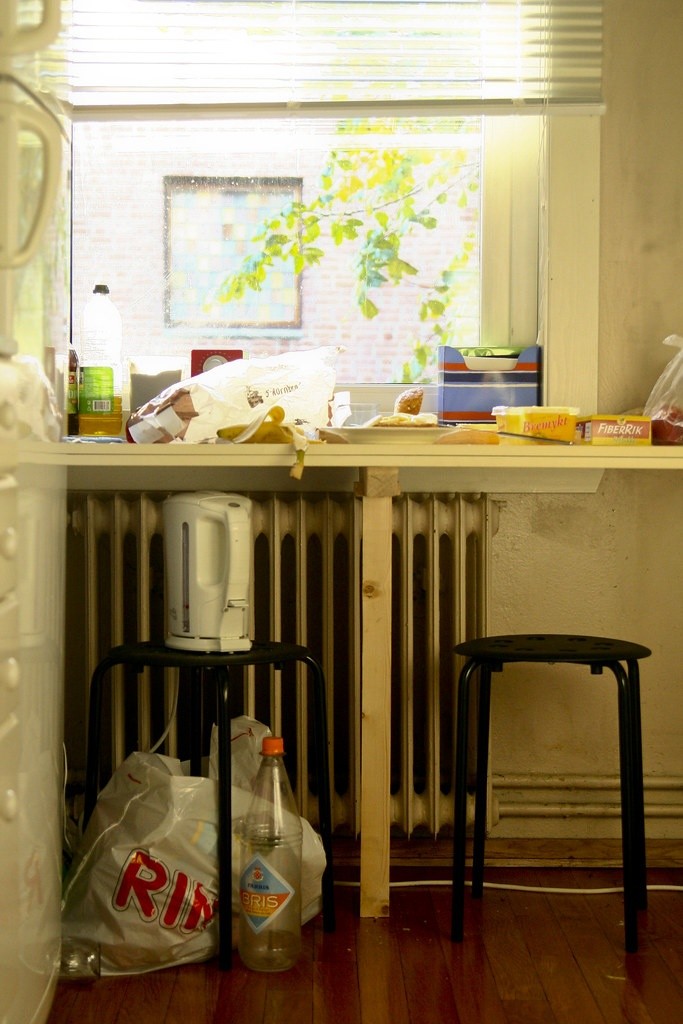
[158,488,259,652]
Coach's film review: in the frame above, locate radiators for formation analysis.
[72,490,499,840]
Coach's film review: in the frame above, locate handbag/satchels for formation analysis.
[126,345,346,445]
[56,717,328,978]
[644,335,683,444]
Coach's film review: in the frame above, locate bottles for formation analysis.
[75,284,134,441]
[238,737,303,973]
[65,345,81,438]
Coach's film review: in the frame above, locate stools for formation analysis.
[82,642,335,972]
[450,635,652,953]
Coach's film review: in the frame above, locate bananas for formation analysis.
[217,403,326,480]
[432,430,499,445]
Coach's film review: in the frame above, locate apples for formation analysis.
[645,405,683,443]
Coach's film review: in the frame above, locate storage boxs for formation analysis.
[437,345,539,422]
[491,405,580,446]
[577,414,652,445]
